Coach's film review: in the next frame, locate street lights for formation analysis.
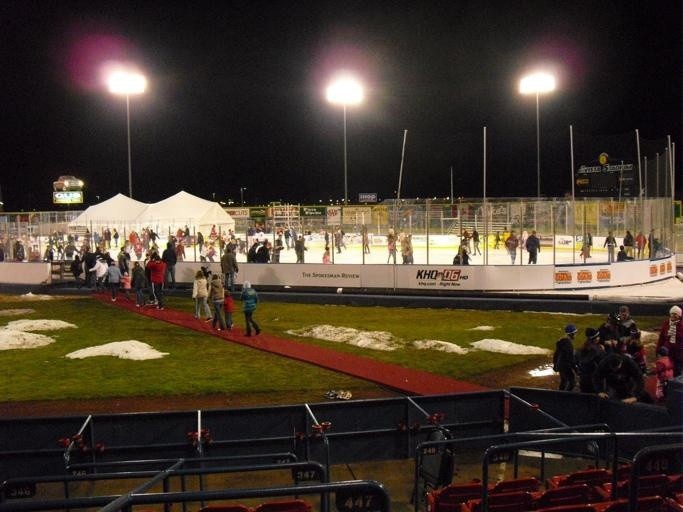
[519,72,554,198]
[104,69,148,199]
[325,77,362,204]
[239,187,248,206]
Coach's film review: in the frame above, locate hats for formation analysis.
[658,346,669,355]
[243,281,251,288]
[565,324,577,334]
[668,305,683,317]
[585,327,599,339]
[619,306,629,313]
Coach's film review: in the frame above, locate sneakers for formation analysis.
[205,317,213,323]
[244,329,260,337]
[111,297,116,302]
[136,300,164,310]
[193,315,200,319]
[209,323,234,331]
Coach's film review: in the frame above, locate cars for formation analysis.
[52,175,86,190]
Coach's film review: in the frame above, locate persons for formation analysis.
[603,231,617,264]
[494,226,529,265]
[579,232,592,259]
[526,231,540,264]
[193,248,261,337]
[452,228,482,266]
[69,243,177,310]
[616,228,661,261]
[552,304,683,405]
[1,223,415,264]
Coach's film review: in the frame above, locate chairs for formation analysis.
[252,499,313,512]
[200,502,248,511]
[426,461,683,512]
